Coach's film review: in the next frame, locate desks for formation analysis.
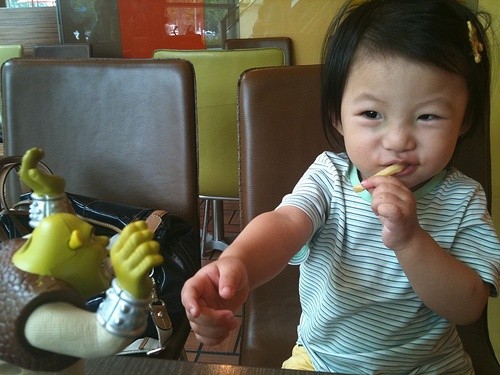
[152,48,286,258]
[51,353,370,375]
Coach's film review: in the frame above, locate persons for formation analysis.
[180,1,499,375]
[0,147,166,372]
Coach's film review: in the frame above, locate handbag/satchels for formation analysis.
[0,154,198,359]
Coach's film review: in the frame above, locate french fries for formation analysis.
[353,164,405,191]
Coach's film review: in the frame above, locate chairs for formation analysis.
[242,66,500,375]
[0,58,192,359]
